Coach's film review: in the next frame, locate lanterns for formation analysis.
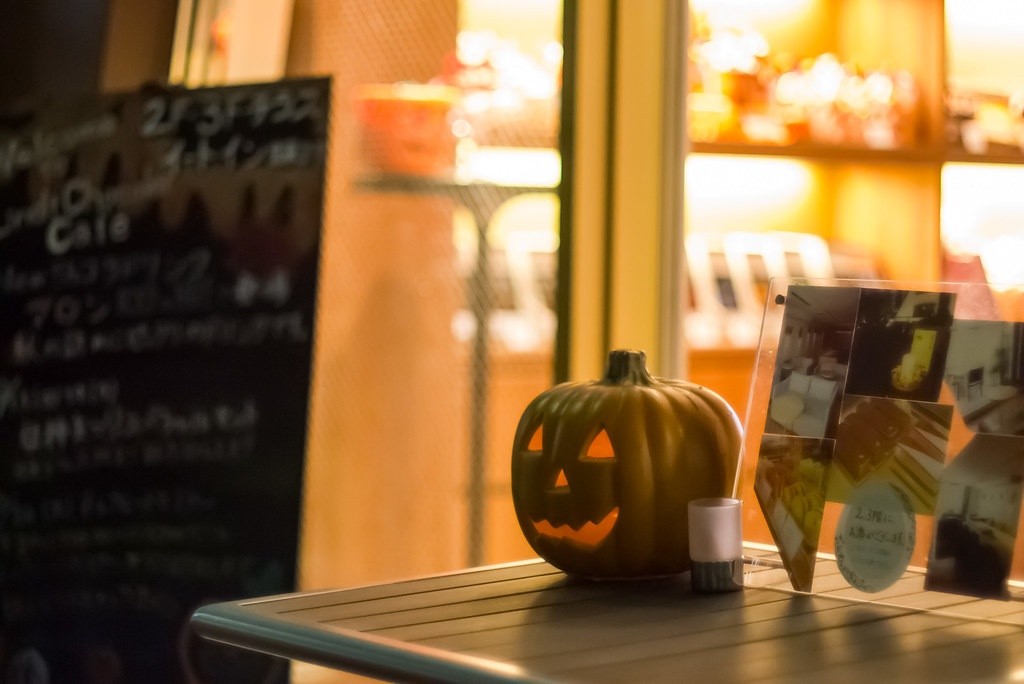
[510,350,742,584]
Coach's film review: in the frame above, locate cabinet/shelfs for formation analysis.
[184,0,1024,581]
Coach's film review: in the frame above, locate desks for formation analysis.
[191,542,1024,684]
